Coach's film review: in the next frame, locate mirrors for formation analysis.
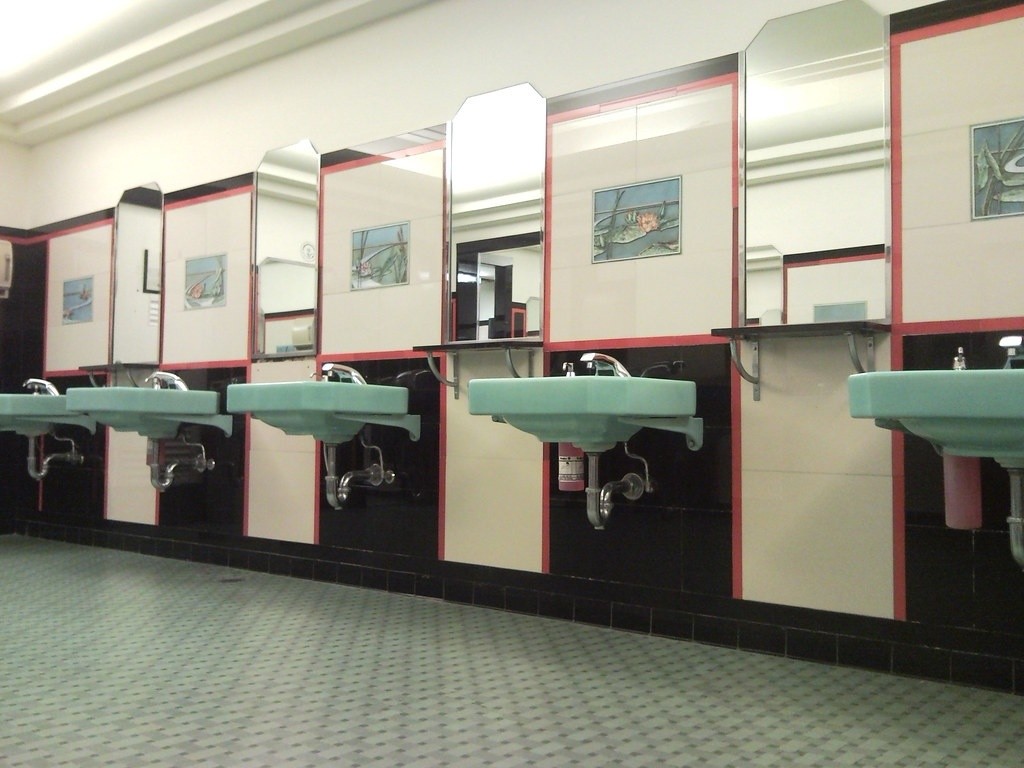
[445,82,547,344]
[736,0,892,338]
[475,244,540,341]
[251,135,321,363]
[110,181,164,368]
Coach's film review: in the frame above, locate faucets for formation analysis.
[322,363,365,384]
[1000,334,1024,362]
[579,353,632,377]
[144,372,189,390]
[22,378,61,395]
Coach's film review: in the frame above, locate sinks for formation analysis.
[0,395,96,436]
[848,368,1024,465]
[227,383,409,446]
[471,376,697,451]
[65,389,220,437]
